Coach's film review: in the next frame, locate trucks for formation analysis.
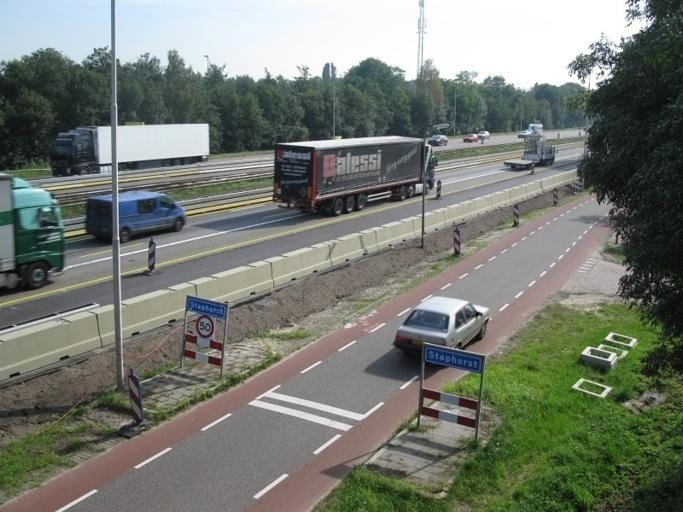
[504,141,555,170]
[50,124,210,177]
[0,174,64,290]
[272,135,438,217]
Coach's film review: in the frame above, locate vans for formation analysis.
[85,190,186,242]
[529,124,544,136]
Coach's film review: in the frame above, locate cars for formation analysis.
[463,131,489,142]
[392,296,490,366]
[429,135,448,146]
[518,130,530,138]
